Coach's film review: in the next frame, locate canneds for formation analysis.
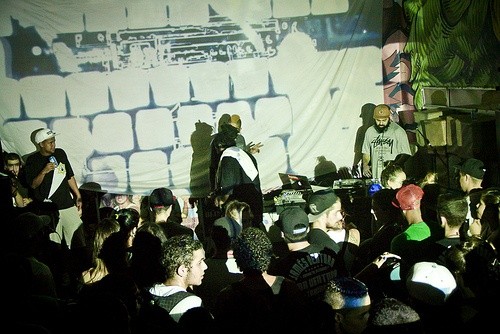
[49,156,58,167]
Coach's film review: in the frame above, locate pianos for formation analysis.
[333,179,381,208]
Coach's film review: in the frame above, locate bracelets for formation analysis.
[472,234,482,237]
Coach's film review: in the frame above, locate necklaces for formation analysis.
[446,232,460,237]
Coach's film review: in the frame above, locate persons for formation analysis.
[21,128,82,248]
[0,153,500,334]
[210,114,263,192]
[214,131,263,229]
[362,104,412,189]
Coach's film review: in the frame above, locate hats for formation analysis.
[228,115,241,130]
[276,207,309,240]
[373,105,391,119]
[18,212,50,237]
[408,261,457,304]
[305,190,337,223]
[35,128,60,144]
[149,187,176,208]
[453,156,486,179]
[392,184,423,211]
